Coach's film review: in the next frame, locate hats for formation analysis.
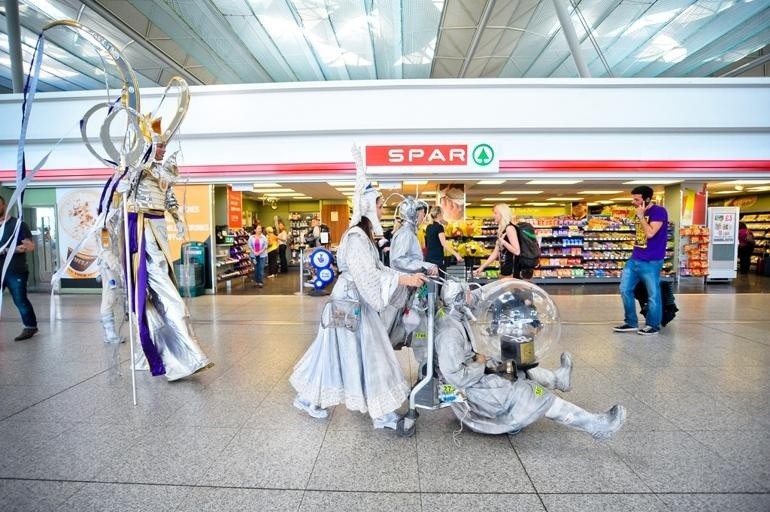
[348,147,384,236]
[431,268,482,319]
[396,198,427,227]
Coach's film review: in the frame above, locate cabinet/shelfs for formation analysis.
[737,214,770,271]
[465,215,711,284]
[216,211,337,287]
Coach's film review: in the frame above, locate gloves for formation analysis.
[175,222,186,241]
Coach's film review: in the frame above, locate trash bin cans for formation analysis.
[173,241,208,296]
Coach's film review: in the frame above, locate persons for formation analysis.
[1,20,214,381]
[739,223,755,274]
[385,192,439,348]
[424,206,462,293]
[612,186,665,335]
[303,216,328,245]
[475,205,520,280]
[99,198,131,344]
[277,223,289,273]
[265,226,278,278]
[0,199,37,340]
[289,143,415,428]
[249,223,268,288]
[436,278,626,441]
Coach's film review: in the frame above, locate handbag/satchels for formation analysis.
[320,298,362,332]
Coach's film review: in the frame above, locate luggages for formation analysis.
[635,276,680,327]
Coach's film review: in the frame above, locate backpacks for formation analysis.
[507,221,542,268]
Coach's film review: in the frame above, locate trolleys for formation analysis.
[385,266,531,440]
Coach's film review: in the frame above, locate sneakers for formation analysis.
[638,326,659,335]
[612,324,638,332]
[105,335,126,344]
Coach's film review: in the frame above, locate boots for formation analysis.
[553,405,626,439]
[554,352,572,393]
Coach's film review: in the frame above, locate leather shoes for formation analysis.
[293,396,329,418]
[14,328,38,342]
[374,412,400,430]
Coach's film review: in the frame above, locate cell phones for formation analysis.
[641,197,651,208]
